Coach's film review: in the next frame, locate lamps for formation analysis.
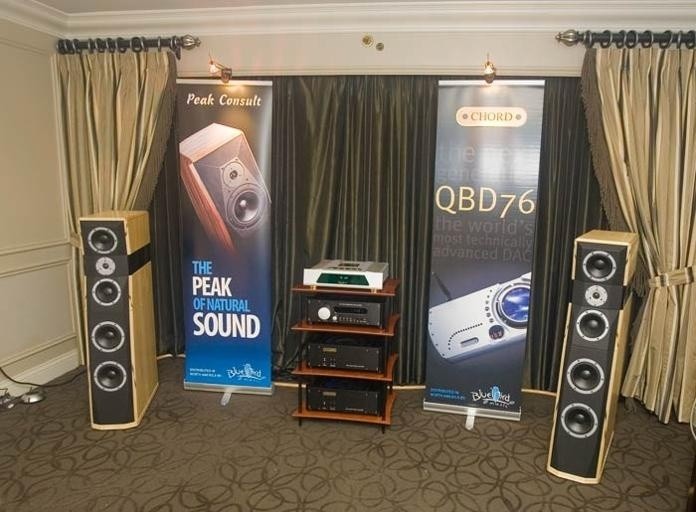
[481,54,502,83]
[206,51,232,82]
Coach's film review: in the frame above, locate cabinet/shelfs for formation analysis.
[289,279,400,432]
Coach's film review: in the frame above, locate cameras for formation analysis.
[426,270,532,363]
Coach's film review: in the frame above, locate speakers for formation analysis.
[179,120,273,254]
[545,229,637,487]
[76,210,160,432]
